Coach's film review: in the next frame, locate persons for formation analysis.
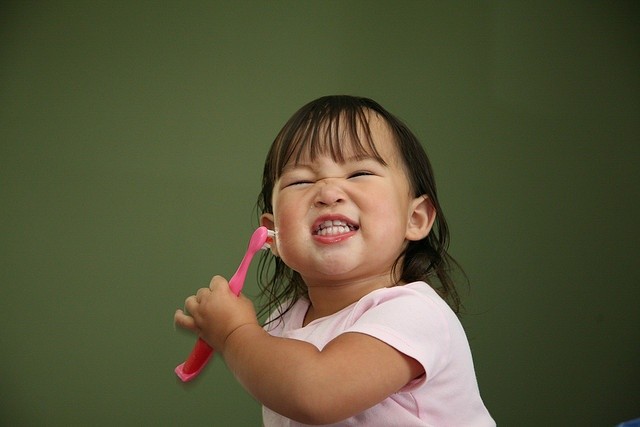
[172,94,498,427]
